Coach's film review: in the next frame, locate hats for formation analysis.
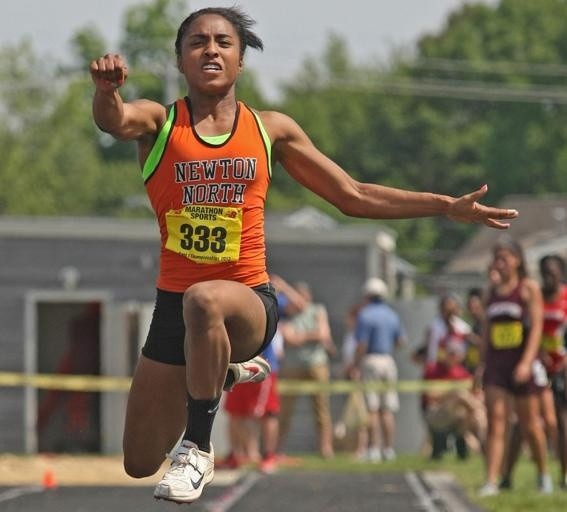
[360,276,388,298]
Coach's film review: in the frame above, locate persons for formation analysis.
[410,241,567,494]
[215,276,407,475]
[91,8,519,503]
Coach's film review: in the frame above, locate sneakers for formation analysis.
[152,438,216,504]
[475,471,566,500]
[367,445,396,464]
[226,354,272,392]
[214,449,280,474]
